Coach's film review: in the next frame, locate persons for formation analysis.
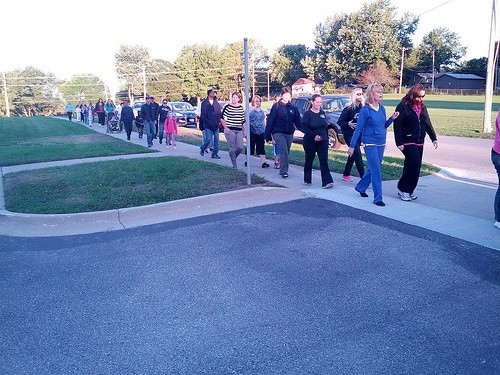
[196,91,218,153]
[65,98,124,131]
[347,83,400,206]
[141,96,171,147]
[244,95,270,168]
[393,84,438,201]
[200,89,224,159]
[265,87,302,178]
[121,100,136,140]
[301,94,334,188]
[491,111,500,229]
[135,111,144,138]
[164,111,177,146]
[273,96,280,169]
[337,86,364,182]
[220,92,246,170]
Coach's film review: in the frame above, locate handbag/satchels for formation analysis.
[199,117,204,131]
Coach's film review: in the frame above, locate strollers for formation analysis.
[107,111,122,135]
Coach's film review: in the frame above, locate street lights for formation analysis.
[128,62,147,103]
[398,46,409,94]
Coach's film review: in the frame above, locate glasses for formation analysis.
[417,95,425,98]
[356,93,363,96]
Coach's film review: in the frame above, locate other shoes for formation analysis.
[373,201,385,206]
[245,161,247,167]
[354,187,368,197]
[262,162,270,168]
[282,172,288,178]
[148,143,151,147]
[322,183,334,188]
[199,148,204,156]
[494,221,500,229]
[274,162,280,168]
[160,141,162,144]
[166,141,171,145]
[151,141,153,146]
[155,136,157,139]
[211,153,221,159]
[304,182,311,186]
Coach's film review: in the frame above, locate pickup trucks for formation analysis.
[266,95,351,149]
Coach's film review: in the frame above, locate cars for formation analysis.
[196,101,228,132]
[167,101,197,127]
[132,101,146,118]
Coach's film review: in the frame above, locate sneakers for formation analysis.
[341,175,353,181]
[410,194,417,200]
[397,187,411,201]
